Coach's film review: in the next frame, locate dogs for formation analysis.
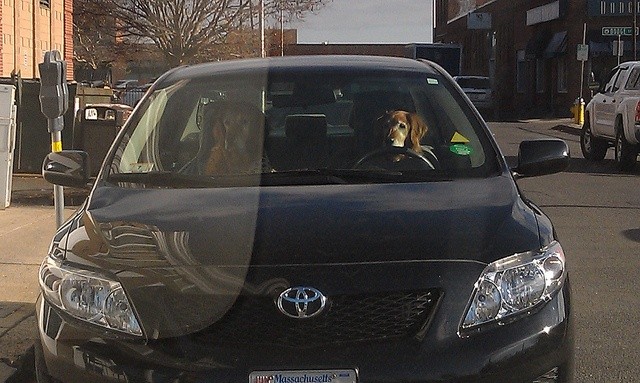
[377,110,429,163]
[205,101,268,174]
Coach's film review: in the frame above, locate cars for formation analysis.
[580,61,640,168]
[34,55,574,383]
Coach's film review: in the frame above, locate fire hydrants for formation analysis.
[570,97,585,126]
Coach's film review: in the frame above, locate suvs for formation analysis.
[453,76,495,120]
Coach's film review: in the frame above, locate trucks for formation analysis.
[404,43,463,77]
[0,77,134,190]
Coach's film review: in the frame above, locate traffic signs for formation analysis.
[602,27,639,36]
[577,44,588,61]
